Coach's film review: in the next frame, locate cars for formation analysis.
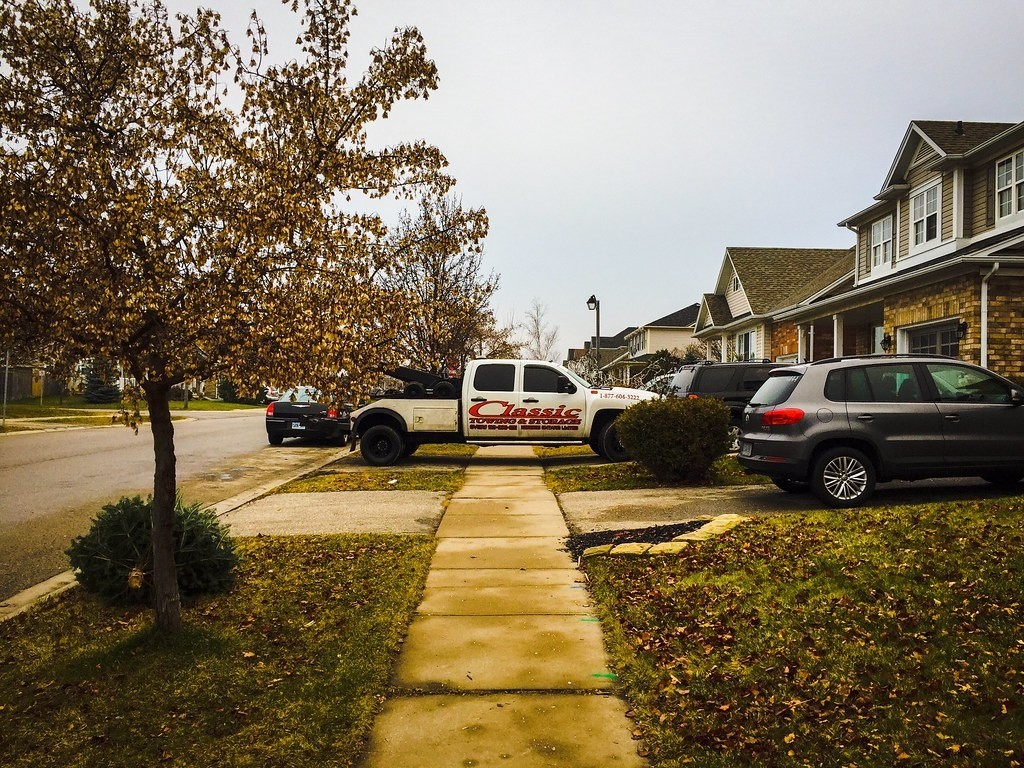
[666,358,802,454]
[265,386,365,447]
[735,352,1024,509]
[644,374,677,396]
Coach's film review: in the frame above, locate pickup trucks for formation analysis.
[346,357,668,463]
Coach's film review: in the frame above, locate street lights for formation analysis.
[586,294,600,386]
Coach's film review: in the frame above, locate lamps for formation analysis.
[881,335,890,352]
[956,322,966,339]
